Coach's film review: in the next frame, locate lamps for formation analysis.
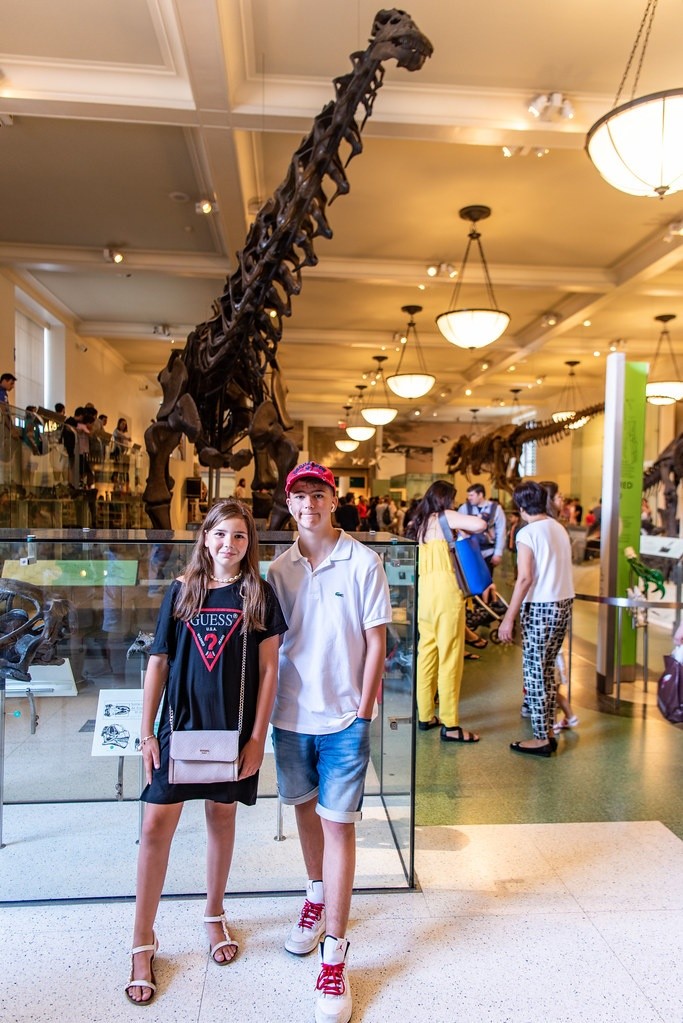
[194,200,217,216]
[335,0,683,453]
[103,246,124,265]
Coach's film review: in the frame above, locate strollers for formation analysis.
[465,590,518,645]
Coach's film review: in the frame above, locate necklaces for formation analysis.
[209,569,243,583]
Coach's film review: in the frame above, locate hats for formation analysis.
[285,461,335,493]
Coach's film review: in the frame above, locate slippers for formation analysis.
[465,636,489,649]
[463,653,482,660]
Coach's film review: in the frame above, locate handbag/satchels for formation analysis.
[657,654,683,724]
[169,730,239,784]
[437,510,494,598]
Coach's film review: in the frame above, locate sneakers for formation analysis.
[284,897,326,954]
[315,939,352,1023]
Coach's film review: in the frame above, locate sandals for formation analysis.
[440,725,480,742]
[124,929,160,1005]
[418,715,440,731]
[203,910,239,965]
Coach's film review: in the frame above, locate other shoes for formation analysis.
[549,738,558,752]
[553,724,560,734]
[77,675,95,693]
[511,740,552,757]
[558,716,578,728]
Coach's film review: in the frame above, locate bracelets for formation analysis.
[140,734,155,744]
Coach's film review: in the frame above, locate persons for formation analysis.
[482,582,505,619]
[497,480,576,758]
[42,536,172,688]
[512,480,579,733]
[265,463,394,1023]
[404,479,488,743]
[640,497,653,534]
[560,496,602,531]
[234,479,246,500]
[464,623,488,661]
[1,373,132,513]
[457,483,506,578]
[335,491,422,536]
[124,501,289,1007]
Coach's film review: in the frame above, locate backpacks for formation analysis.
[382,506,392,525]
[467,503,498,550]
